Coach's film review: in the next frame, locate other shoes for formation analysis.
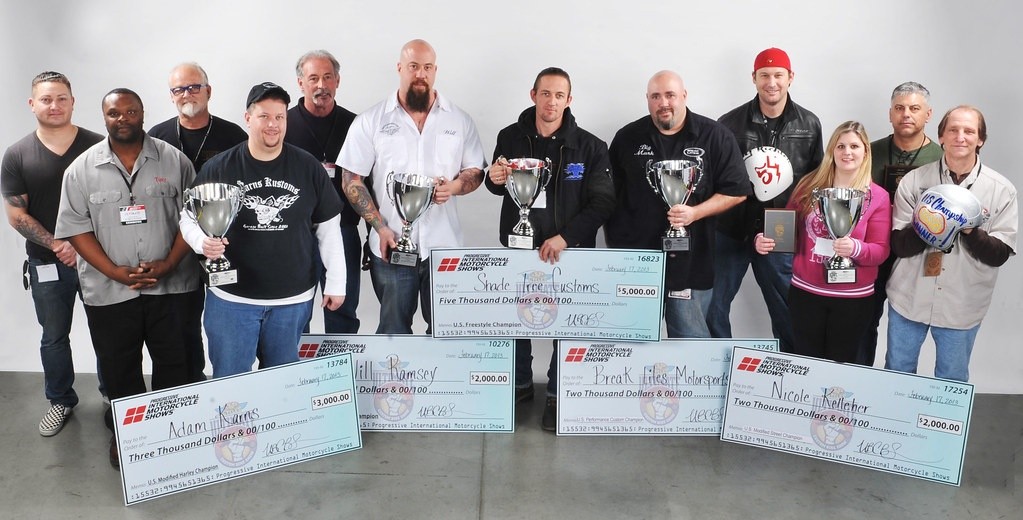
[39,402,70,436]
[102,394,111,404]
[109,433,120,466]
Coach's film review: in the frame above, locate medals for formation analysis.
[762,208,798,255]
[883,164,919,206]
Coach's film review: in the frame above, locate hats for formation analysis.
[754,48,791,71]
[246,82,291,107]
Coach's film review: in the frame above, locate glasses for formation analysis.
[23,261,31,291]
[171,84,208,96]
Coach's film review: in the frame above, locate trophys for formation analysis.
[811,186,871,285]
[495,155,553,250]
[645,157,702,253]
[184,180,245,287]
[385,170,444,267]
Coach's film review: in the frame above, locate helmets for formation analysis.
[911,185,982,251]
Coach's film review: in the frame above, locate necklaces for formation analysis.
[939,156,982,191]
[889,134,926,167]
[176,114,213,166]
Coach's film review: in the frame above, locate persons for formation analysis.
[704,47,824,354]
[333,39,488,335]
[484,67,617,431]
[883,106,1017,383]
[54,87,207,471]
[282,49,376,335]
[145,63,256,209]
[755,121,892,367]
[179,82,346,380]
[1,71,111,437]
[603,70,753,338]
